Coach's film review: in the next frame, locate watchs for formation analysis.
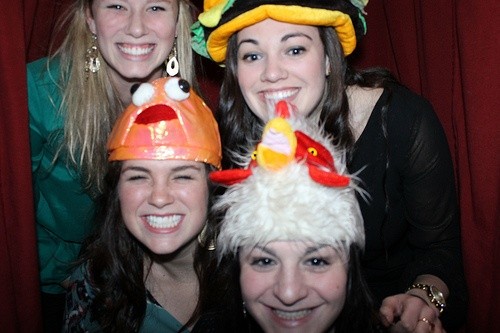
[409,283,447,312]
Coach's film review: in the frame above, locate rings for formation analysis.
[418,318,435,333]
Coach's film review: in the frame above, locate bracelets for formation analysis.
[406,292,437,316]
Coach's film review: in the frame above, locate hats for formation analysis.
[190,0,369,67]
[207,97,373,269]
[105,75,223,171]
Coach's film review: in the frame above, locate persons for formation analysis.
[207,101,410,333]
[59,75,244,333]
[190,0,465,333]
[24,0,209,333]
